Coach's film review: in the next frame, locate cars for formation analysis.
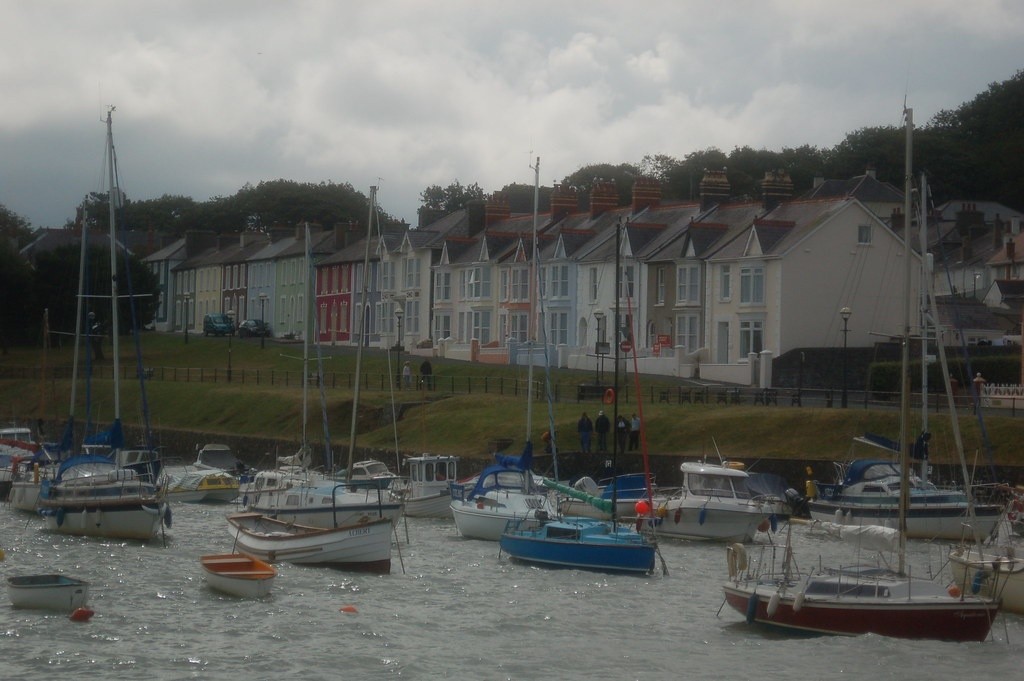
[237,318,274,339]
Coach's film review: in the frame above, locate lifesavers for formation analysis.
[33,462,39,483]
[728,542,748,576]
[605,388,614,404]
[1008,499,1022,524]
[728,462,745,469]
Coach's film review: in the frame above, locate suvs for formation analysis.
[202,314,235,337]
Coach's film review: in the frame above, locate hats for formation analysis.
[599,411,604,416]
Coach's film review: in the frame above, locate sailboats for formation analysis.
[0,104,175,538]
[719,92,1024,643]
[230,181,682,520]
[498,212,664,576]
[783,169,1015,544]
[449,147,567,544]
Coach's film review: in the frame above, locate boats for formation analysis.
[197,551,279,598]
[224,474,396,575]
[941,449,1024,618]
[155,465,242,504]
[632,434,793,548]
[191,440,245,476]
[6,572,92,615]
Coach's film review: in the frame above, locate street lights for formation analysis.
[85,311,96,375]
[592,309,606,384]
[839,306,854,409]
[394,307,406,387]
[258,292,269,349]
[183,291,191,344]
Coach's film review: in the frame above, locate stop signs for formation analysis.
[620,340,632,353]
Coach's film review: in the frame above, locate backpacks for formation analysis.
[618,418,625,428]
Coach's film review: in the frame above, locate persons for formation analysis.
[87,311,105,360]
[578,411,640,451]
[420,359,433,390]
[542,430,559,453]
[402,361,412,389]
